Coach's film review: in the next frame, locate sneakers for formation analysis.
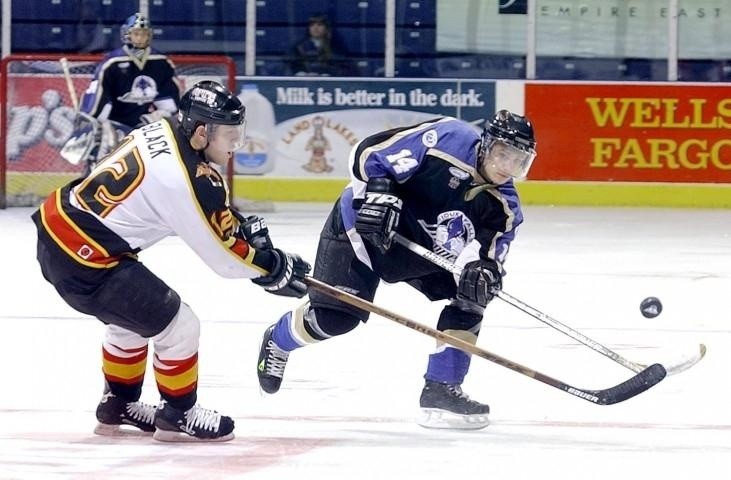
[258,324,290,393]
[156,397,234,438]
[420,380,489,414]
[96,379,158,432]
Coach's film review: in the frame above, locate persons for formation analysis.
[258,110,539,415]
[285,14,351,78]
[31,81,312,444]
[70,11,183,178]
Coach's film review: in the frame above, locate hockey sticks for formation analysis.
[384,227,708,378]
[285,266,667,408]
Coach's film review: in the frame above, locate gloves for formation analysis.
[251,247,312,298]
[457,260,502,307]
[235,215,273,251]
[356,177,405,255]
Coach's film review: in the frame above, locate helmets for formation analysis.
[480,110,537,178]
[180,81,247,152]
[120,13,153,49]
